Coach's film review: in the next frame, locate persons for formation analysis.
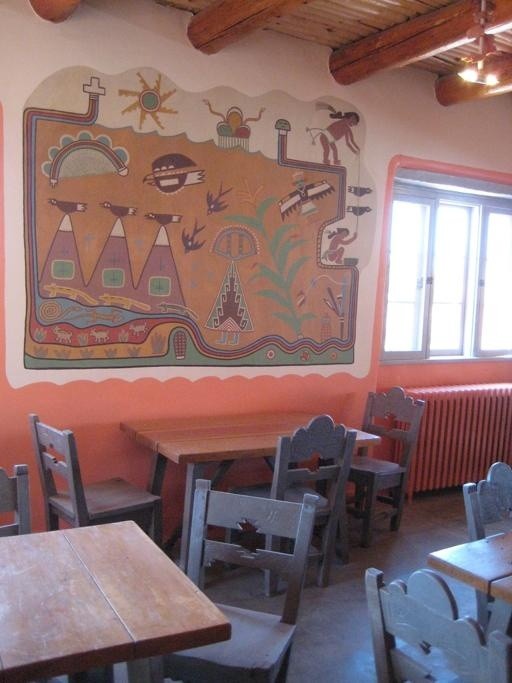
[319,111,361,165]
[328,227,358,265]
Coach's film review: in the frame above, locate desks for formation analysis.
[112,412,387,576]
[422,526,510,605]
[1,515,235,680]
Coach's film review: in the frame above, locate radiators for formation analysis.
[382,384,511,505]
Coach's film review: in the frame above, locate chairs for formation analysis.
[324,385,433,549]
[456,457,509,632]
[361,562,509,681]
[223,409,360,599]
[145,476,321,680]
[27,411,167,557]
[0,459,38,537]
